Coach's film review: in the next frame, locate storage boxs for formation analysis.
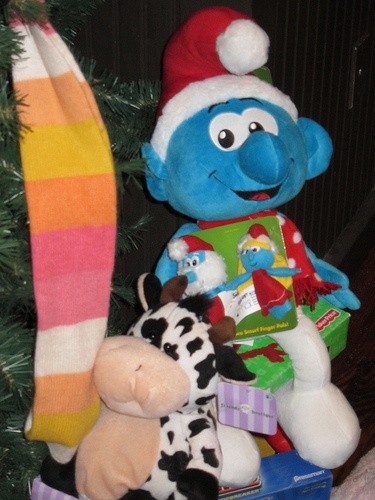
[215,447,333,499]
[221,296,351,397]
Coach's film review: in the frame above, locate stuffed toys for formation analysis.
[136,5,362,469]
[230,223,301,320]
[124,271,259,500]
[74,332,190,500]
[164,223,238,324]
